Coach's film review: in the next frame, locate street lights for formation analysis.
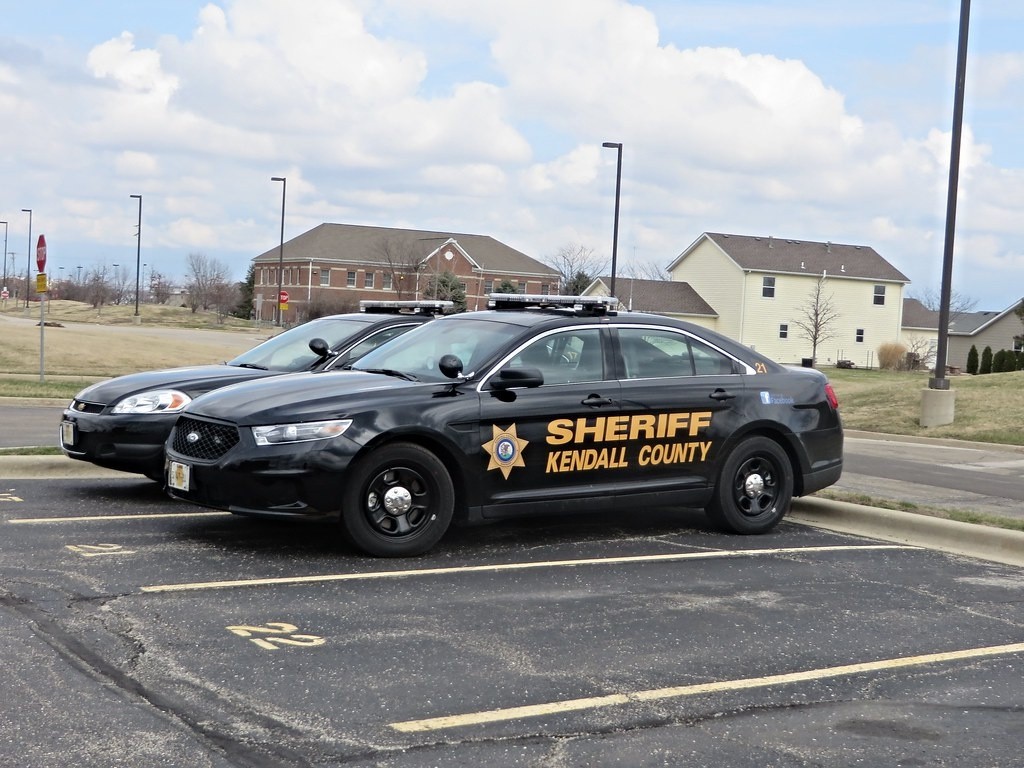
[130,194,142,315]
[113,264,120,300]
[57,267,64,285]
[22,209,32,307]
[271,177,286,325]
[603,142,623,310]
[142,264,147,295]
[77,266,83,283]
[1,221,7,287]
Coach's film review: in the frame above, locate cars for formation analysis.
[161,287,843,558]
[56,300,580,483]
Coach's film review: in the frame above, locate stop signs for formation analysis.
[1,292,9,299]
[277,291,289,303]
[37,235,46,272]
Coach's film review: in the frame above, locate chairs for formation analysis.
[465,334,511,377]
[569,335,641,383]
